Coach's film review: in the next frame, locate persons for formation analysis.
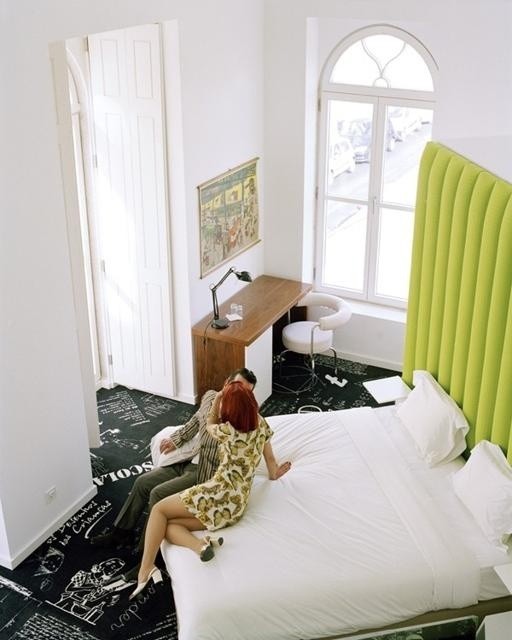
[126,380,293,604]
[86,366,259,573]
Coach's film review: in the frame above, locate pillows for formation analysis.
[393,369,510,549]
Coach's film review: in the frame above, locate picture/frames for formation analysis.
[196,156,262,280]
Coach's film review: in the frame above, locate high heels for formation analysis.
[199,535,224,562]
[128,567,164,601]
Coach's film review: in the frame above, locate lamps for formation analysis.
[208,267,255,329]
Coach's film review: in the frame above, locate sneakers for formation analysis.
[126,551,143,562]
[89,527,131,546]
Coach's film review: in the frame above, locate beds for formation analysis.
[149,401,512,638]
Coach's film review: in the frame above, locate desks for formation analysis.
[190,276,313,414]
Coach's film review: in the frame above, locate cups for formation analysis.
[230,303,244,320]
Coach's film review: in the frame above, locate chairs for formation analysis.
[271,291,352,401]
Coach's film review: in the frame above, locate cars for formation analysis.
[330,107,431,179]
